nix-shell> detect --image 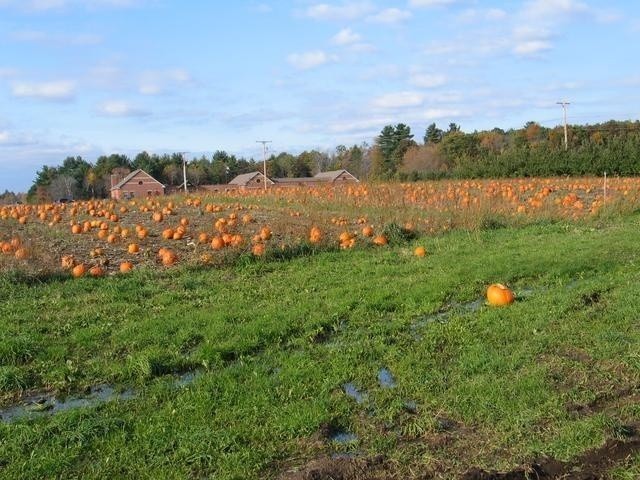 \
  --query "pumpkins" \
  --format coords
[0,176,640,277]
[487,282,513,304]
